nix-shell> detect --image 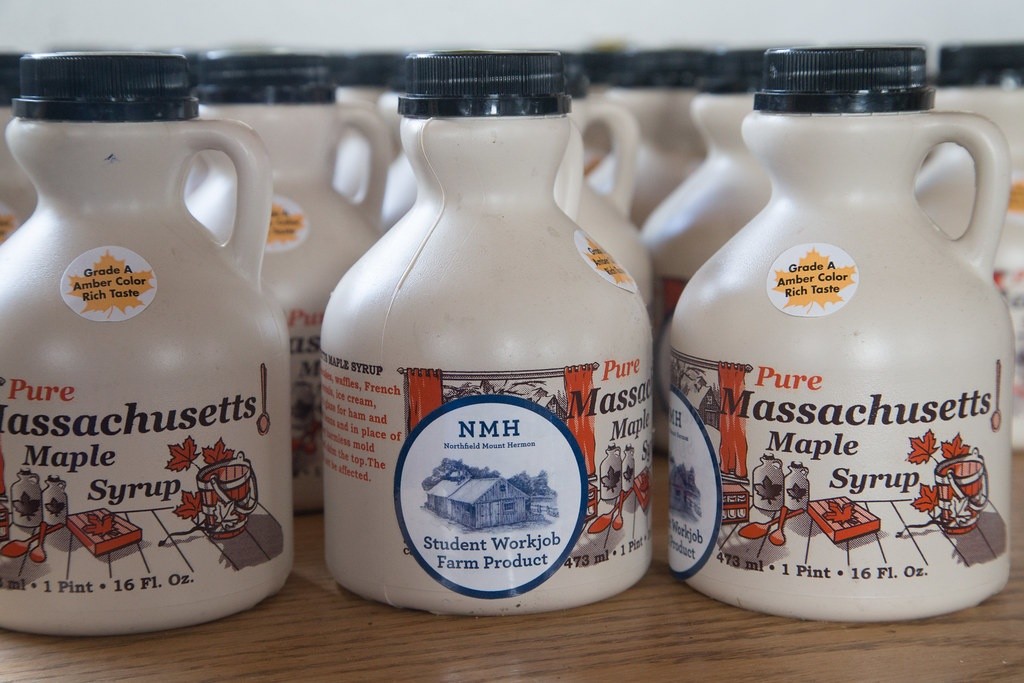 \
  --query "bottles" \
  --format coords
[1,43,1024,638]
